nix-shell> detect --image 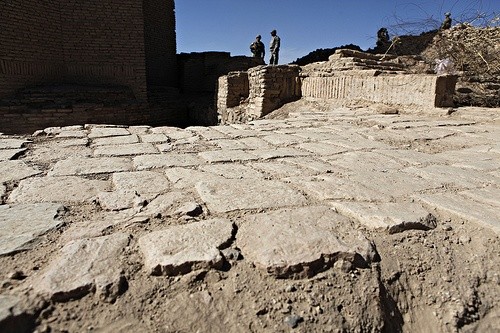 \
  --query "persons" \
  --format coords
[439,13,452,29]
[250,34,266,59]
[268,30,281,65]
[376,27,390,47]
